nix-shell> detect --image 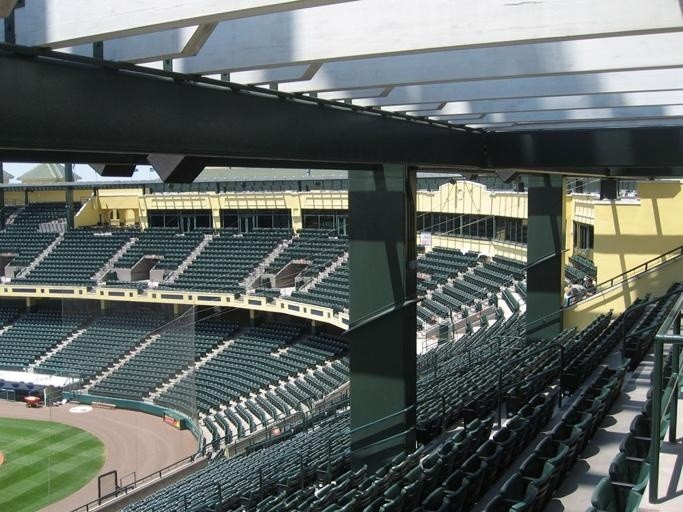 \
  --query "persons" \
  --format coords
[564,275,596,306]
